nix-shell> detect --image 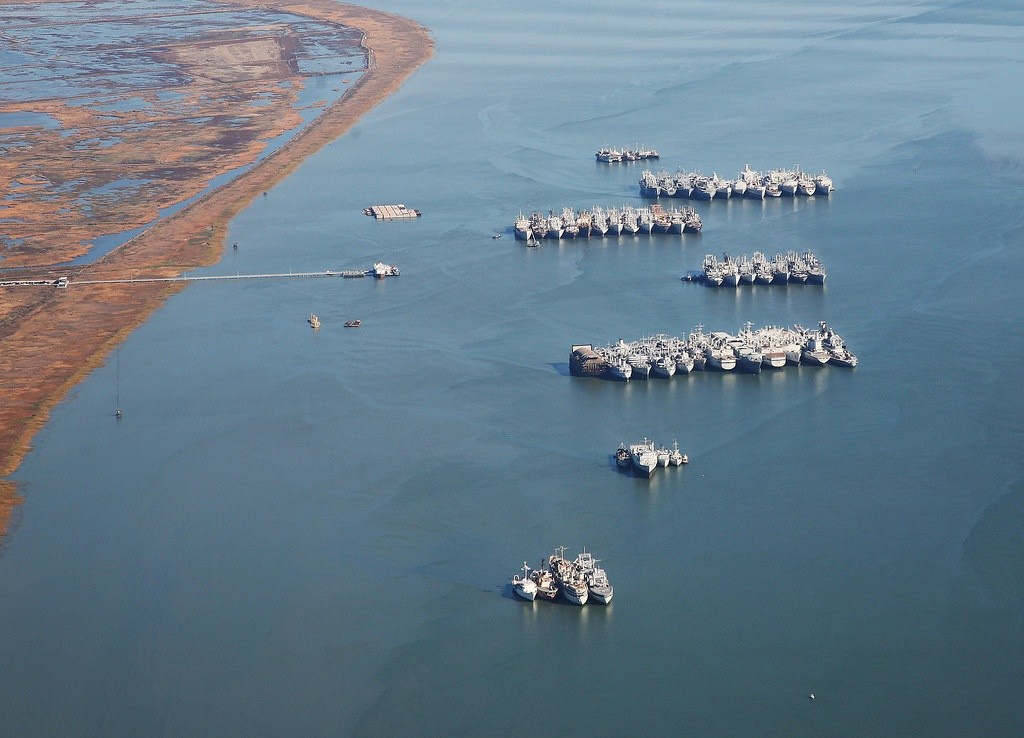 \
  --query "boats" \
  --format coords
[614,433,690,479]
[639,163,836,199]
[594,143,660,162]
[363,261,400,279]
[513,203,703,241]
[568,320,858,378]
[308,314,321,328]
[701,247,828,288]
[343,319,361,328]
[511,545,614,605]
